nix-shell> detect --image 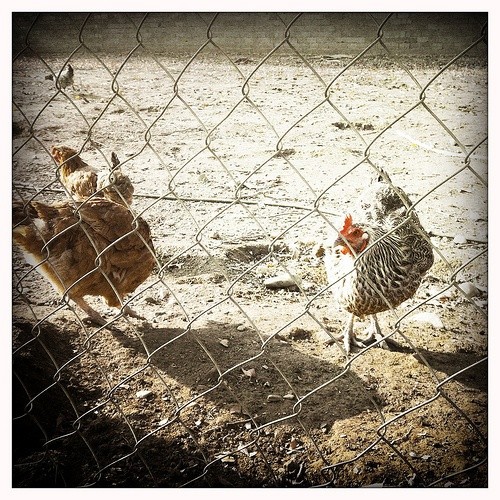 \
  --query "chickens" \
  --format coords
[44,63,77,92]
[12,198,162,332]
[50,143,134,206]
[322,166,436,355]
